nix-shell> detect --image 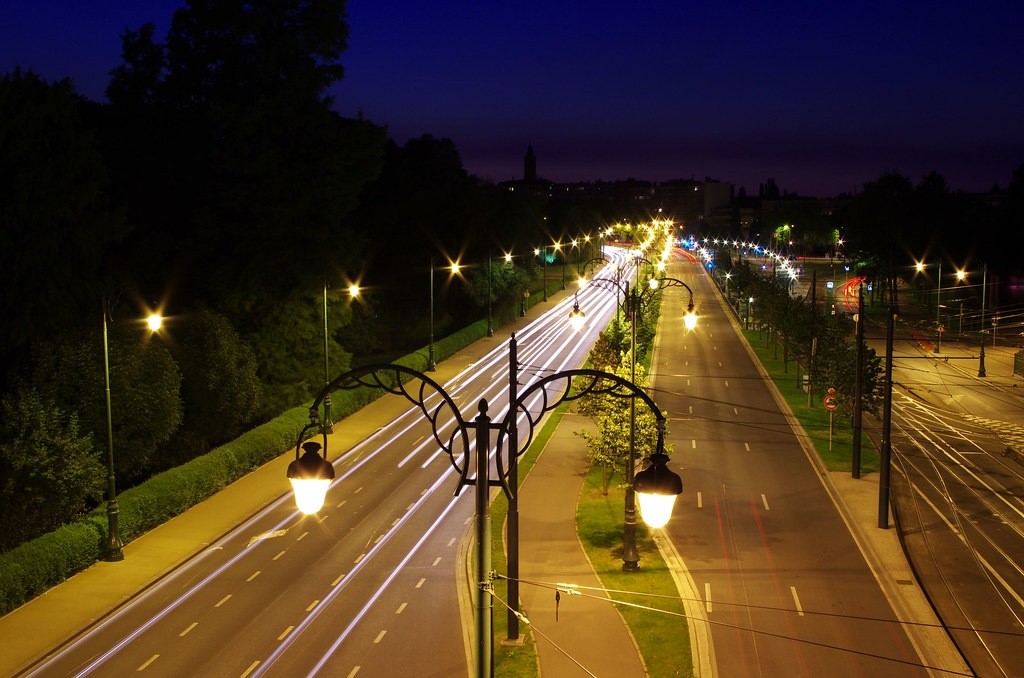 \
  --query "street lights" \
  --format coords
[562,240,577,291]
[102,311,163,562]
[915,259,939,354]
[520,247,542,317]
[485,252,513,338]
[319,283,359,434]
[575,256,659,331]
[426,254,462,372]
[568,277,698,572]
[954,264,986,378]
[286,332,684,678]
[542,243,562,302]
[577,236,590,280]
